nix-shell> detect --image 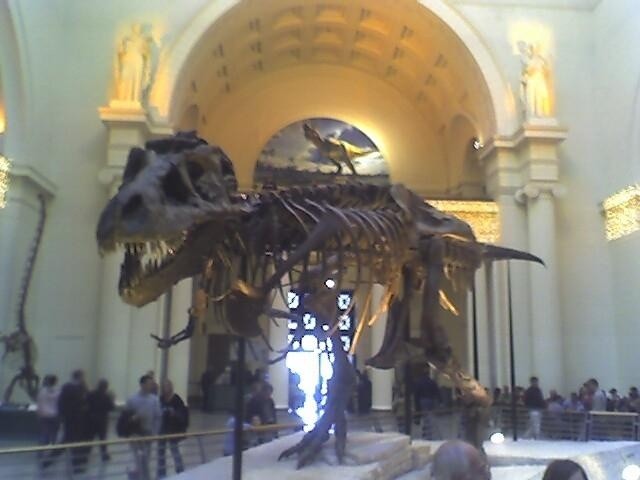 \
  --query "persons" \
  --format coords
[38,371,189,479]
[200,369,324,457]
[392,369,639,443]
[431,438,492,479]
[346,371,373,415]
[541,458,590,480]
